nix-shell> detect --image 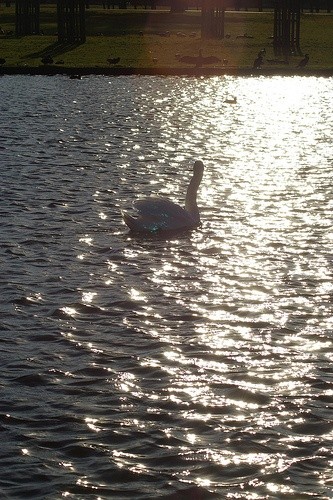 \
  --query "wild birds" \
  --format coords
[122,160,204,231]
